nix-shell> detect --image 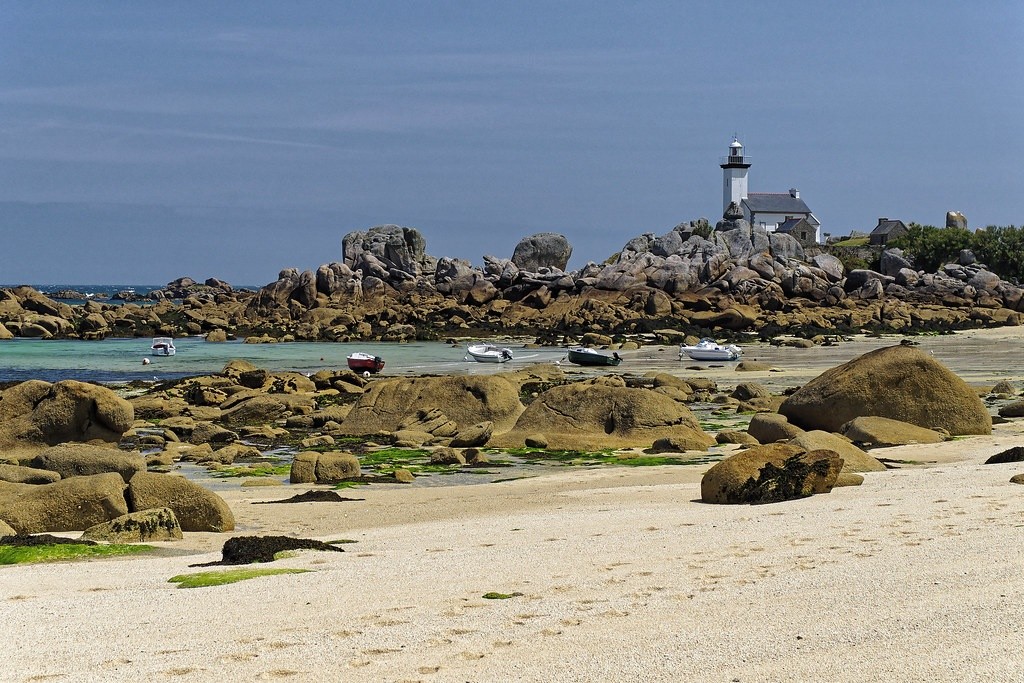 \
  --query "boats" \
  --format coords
[346,352,386,374]
[567,342,624,366]
[150,336,176,357]
[467,343,514,364]
[679,336,745,362]
